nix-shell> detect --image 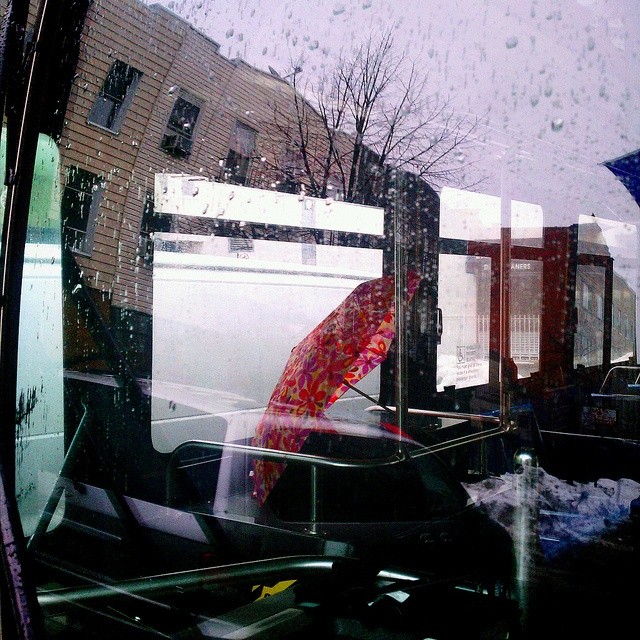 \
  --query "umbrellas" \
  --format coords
[249,267,424,507]
